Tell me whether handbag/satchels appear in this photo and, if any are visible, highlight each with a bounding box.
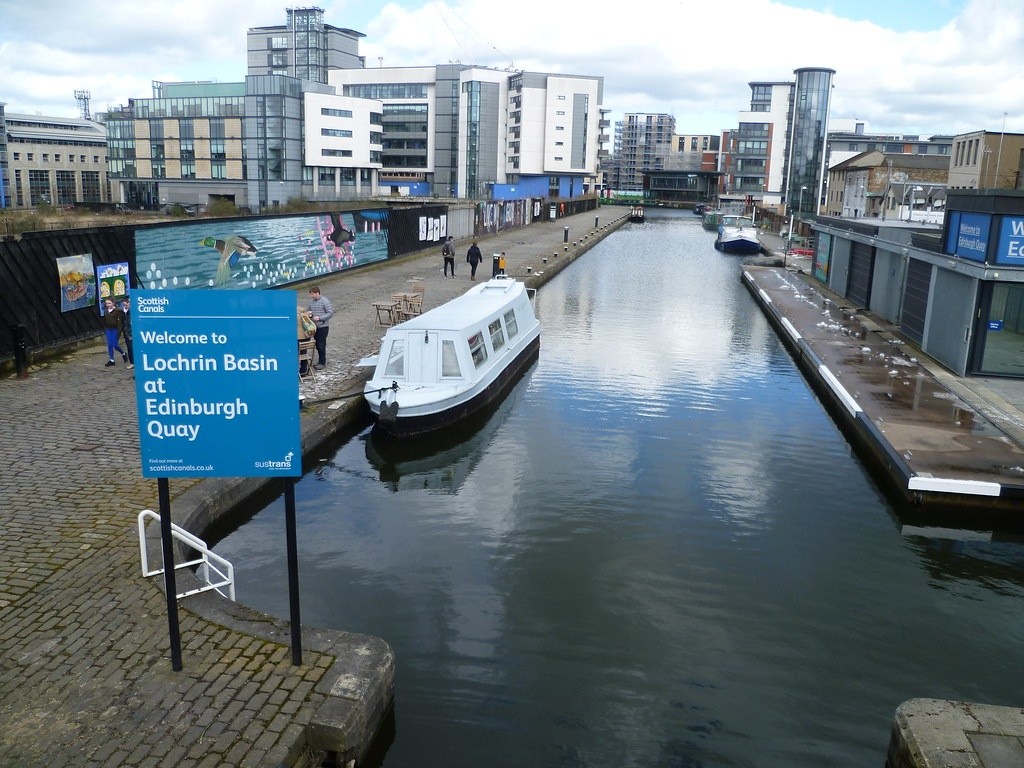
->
[302,314,316,335]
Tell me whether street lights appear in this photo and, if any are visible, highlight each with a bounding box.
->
[799,186,807,213]
[788,210,794,241]
[761,184,766,194]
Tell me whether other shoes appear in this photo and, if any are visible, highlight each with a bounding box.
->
[444,277,448,279]
[127,364,134,369]
[300,372,307,377]
[451,275,456,278]
[122,352,128,362]
[105,361,115,367]
[313,363,326,370]
[471,276,476,281]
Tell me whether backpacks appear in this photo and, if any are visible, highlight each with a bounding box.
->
[442,243,452,256]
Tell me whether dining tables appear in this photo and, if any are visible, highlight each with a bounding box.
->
[372,301,400,331]
[393,293,419,320]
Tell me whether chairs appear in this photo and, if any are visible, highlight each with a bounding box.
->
[299,340,316,384]
[411,286,425,314]
[404,298,423,322]
[388,295,403,325]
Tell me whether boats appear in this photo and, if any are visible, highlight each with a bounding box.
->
[693,203,707,215]
[714,215,762,255]
[364,274,539,439]
[701,206,725,230]
[628,206,645,223]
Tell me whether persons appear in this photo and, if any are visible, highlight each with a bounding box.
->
[297,286,333,377]
[497,251,506,279]
[442,235,457,280]
[466,241,483,281]
[102,295,135,369]
